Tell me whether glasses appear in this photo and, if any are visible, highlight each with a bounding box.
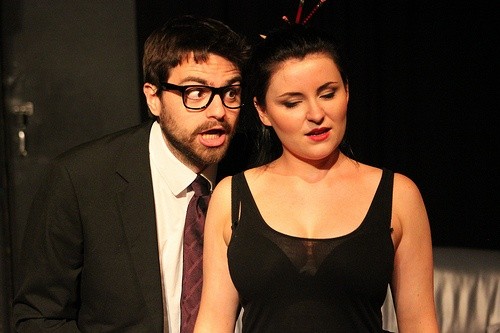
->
[162,80,249,110]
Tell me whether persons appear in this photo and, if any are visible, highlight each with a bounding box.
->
[192,24,437,333]
[8,14,276,333]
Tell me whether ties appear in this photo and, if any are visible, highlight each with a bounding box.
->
[179,175,211,333]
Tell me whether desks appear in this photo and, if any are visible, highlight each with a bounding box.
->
[380,247,500,333]
